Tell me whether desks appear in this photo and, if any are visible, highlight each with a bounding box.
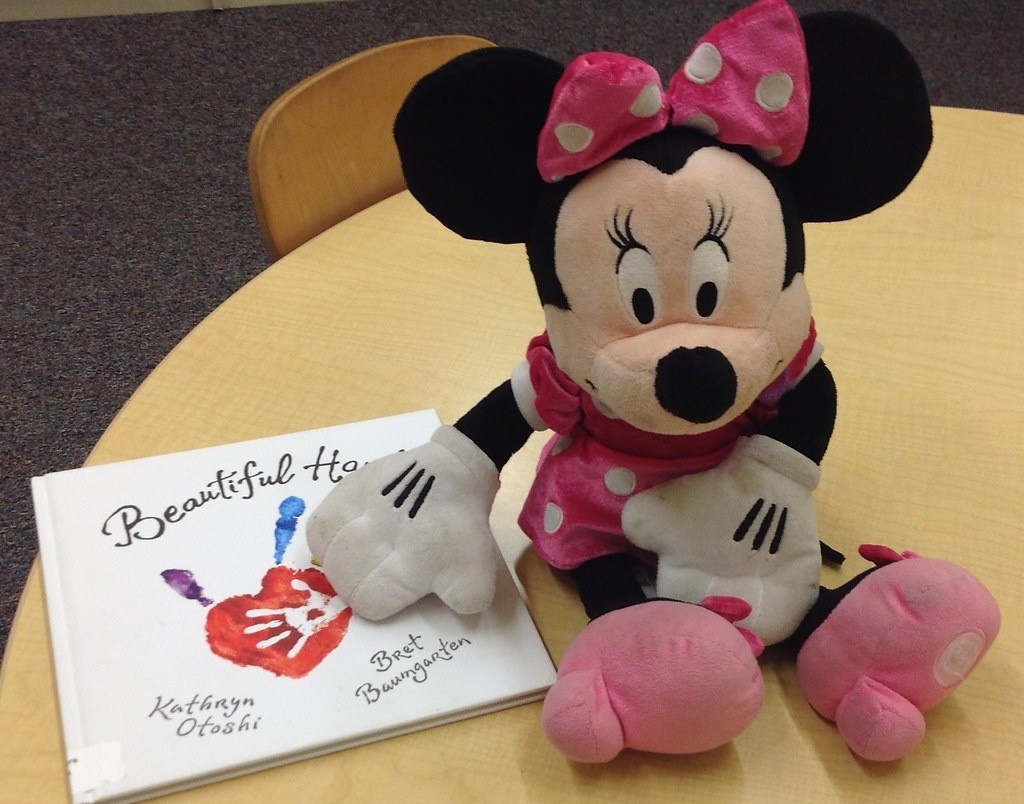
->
[2,101,1024,804]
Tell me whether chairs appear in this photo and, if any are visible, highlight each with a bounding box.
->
[254,34,495,262]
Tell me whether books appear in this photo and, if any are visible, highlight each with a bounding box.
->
[29,409,559,804]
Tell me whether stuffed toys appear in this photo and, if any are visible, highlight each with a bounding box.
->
[307,0,1000,765]
